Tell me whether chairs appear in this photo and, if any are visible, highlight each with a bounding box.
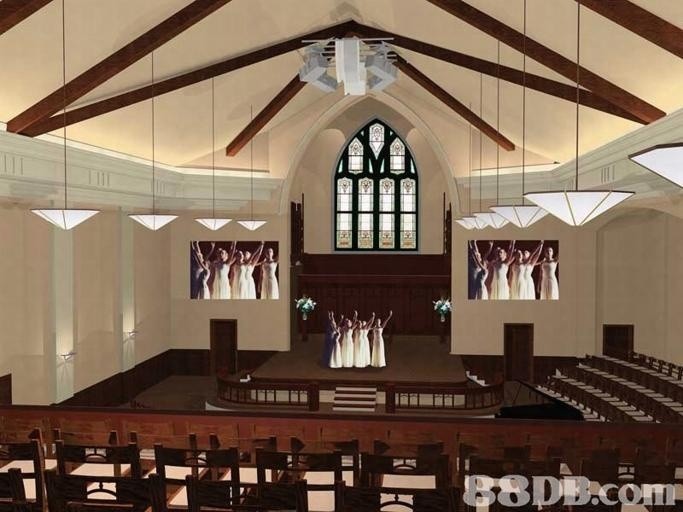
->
[512,348,682,419]
[1,422,681,512]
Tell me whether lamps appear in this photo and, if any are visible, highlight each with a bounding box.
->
[630,143,682,189]
[522,1,636,228]
[298,31,400,101]
[32,1,98,230]
[236,103,267,232]
[193,77,234,231]
[456,40,507,230]
[127,51,179,232]
[488,1,549,229]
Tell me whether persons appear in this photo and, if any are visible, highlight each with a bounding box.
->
[324,309,393,369]
[468,239,559,300]
[190,240,279,300]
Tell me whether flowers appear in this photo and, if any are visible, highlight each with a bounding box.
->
[292,294,318,319]
[431,294,453,319]
